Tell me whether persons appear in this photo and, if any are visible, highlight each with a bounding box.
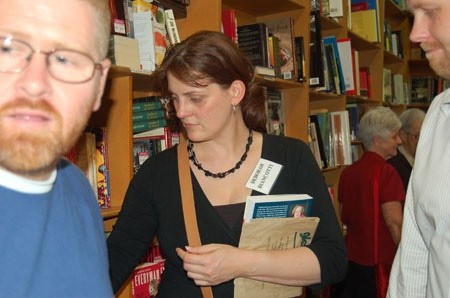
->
[0,0,114,298]
[387,0,450,298]
[337,108,405,298]
[387,108,427,194]
[107,30,348,298]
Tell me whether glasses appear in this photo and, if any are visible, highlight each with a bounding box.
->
[0,35,103,84]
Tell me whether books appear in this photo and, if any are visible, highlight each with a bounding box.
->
[64,0,450,298]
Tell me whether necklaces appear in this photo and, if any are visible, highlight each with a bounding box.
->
[186,128,253,178]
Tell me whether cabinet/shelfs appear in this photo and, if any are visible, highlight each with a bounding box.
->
[62,0,411,298]
[407,12,449,109]
